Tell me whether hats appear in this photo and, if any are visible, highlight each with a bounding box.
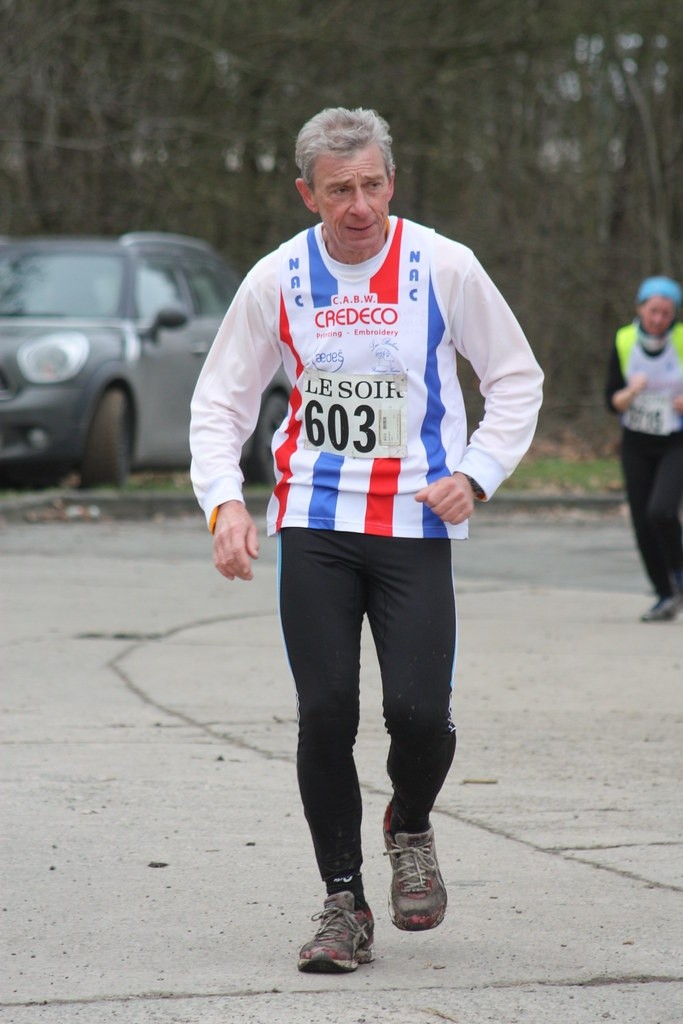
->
[636,275,682,306]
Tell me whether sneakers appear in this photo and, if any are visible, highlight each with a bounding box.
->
[298,891,375,971]
[382,801,447,932]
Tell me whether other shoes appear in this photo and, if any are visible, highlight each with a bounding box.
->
[641,590,683,621]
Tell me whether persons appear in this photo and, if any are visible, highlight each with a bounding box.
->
[189,108,545,973]
[604,277,683,624]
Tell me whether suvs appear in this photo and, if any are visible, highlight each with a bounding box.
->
[1,226,298,494]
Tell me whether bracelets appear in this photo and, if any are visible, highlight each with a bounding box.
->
[467,476,483,496]
